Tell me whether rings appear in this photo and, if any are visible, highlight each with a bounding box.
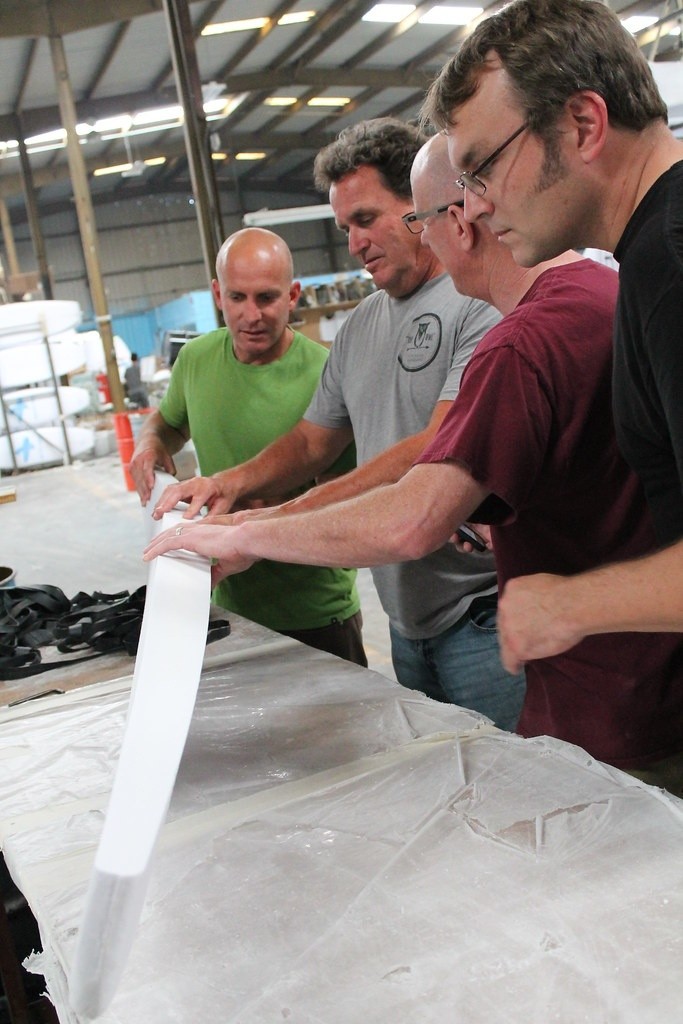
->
[175,526,184,536]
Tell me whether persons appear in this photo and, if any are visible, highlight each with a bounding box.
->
[420,1,683,677]
[127,225,369,669]
[142,129,683,800]
[153,113,526,734]
[123,352,151,410]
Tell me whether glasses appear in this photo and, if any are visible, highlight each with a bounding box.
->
[402,200,464,234]
[454,123,532,196]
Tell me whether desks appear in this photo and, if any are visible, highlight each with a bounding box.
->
[0,463,683,1024]
